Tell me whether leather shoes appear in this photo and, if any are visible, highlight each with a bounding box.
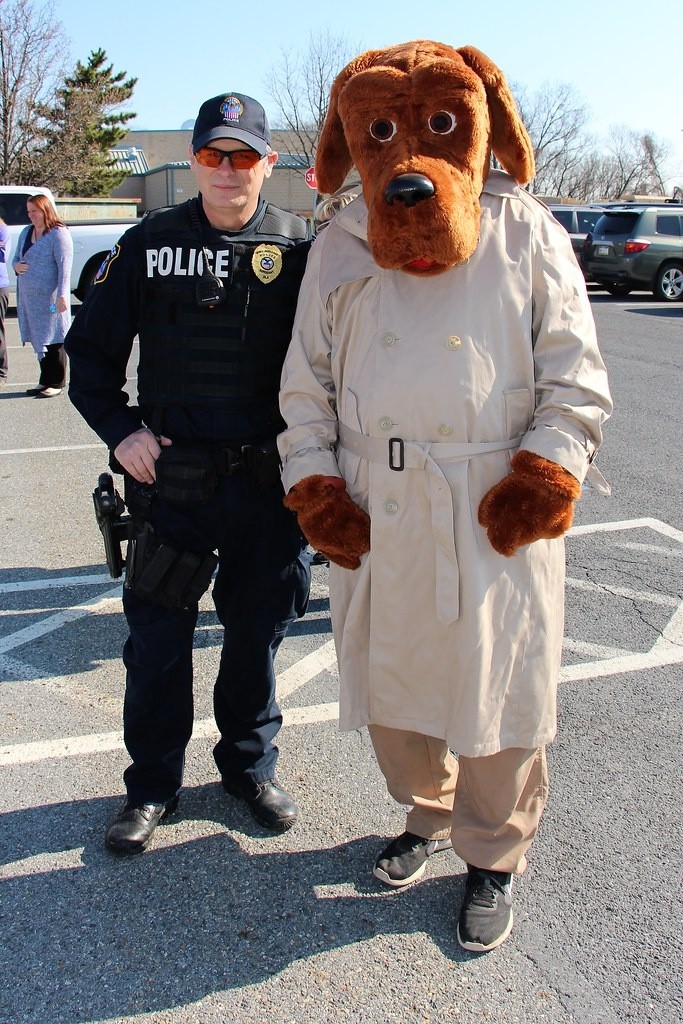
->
[105,789,178,854]
[221,772,300,832]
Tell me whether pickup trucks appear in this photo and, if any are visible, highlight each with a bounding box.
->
[1,185,145,303]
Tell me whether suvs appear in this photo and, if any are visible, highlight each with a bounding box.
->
[544,206,606,282]
[582,202,683,302]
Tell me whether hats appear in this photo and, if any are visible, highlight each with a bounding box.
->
[190,92,271,159]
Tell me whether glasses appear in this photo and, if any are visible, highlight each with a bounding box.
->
[194,145,262,169]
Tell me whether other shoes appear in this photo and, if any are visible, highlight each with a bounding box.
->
[26,384,47,396]
[0,377,7,387]
[39,387,62,398]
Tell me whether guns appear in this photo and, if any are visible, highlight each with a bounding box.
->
[95,472,127,579]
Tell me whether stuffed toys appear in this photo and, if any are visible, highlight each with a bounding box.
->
[278,40,613,953]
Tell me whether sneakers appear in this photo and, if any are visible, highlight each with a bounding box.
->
[457,862,514,951]
[371,831,455,886]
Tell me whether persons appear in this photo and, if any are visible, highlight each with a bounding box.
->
[0,218,10,381]
[62,91,317,851]
[11,194,73,397]
